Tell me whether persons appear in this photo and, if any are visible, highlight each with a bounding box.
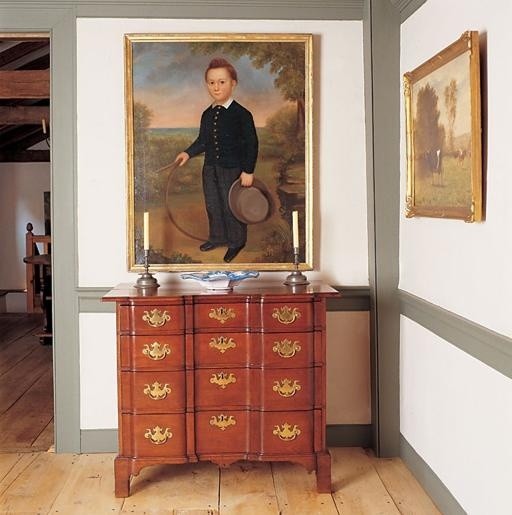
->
[175,56,260,263]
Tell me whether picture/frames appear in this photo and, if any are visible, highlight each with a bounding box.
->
[402,30,484,224]
[123,32,314,272]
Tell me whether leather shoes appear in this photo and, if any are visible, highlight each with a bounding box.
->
[224,245,246,263]
[200,242,225,252]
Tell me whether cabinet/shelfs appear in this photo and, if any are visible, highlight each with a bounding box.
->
[101,282,341,498]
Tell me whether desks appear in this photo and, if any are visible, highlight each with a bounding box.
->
[24,254,52,345]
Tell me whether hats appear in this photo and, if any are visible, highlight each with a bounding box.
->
[229,179,274,225]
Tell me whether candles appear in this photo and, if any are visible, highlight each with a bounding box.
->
[143,211,150,251]
[292,210,299,248]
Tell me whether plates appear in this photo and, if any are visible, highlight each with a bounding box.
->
[179,268,260,291]
